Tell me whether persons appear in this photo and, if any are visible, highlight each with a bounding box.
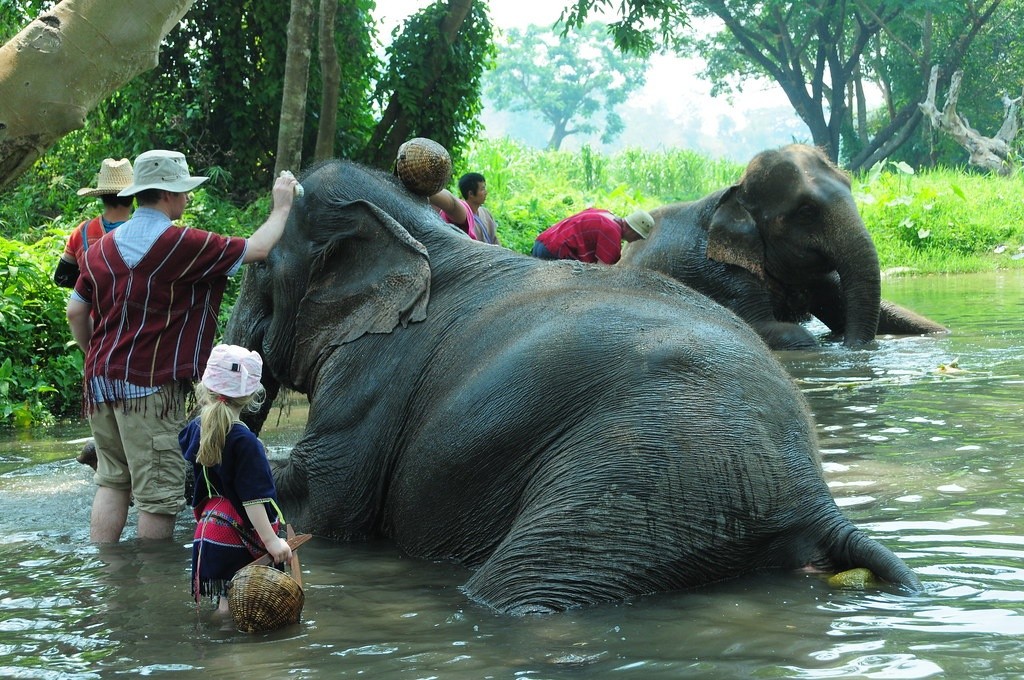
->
[54,159,135,290]
[177,343,293,613]
[427,188,479,243]
[458,172,499,246]
[529,206,655,266]
[67,149,299,542]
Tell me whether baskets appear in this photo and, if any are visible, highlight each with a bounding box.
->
[392,137,452,198]
[224,565,304,634]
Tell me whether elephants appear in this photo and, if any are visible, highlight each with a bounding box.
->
[616,143,948,349]
[76,155,928,620]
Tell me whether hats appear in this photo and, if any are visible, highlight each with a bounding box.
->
[117,150,210,196]
[624,211,655,239]
[202,344,263,398]
[77,158,136,196]
